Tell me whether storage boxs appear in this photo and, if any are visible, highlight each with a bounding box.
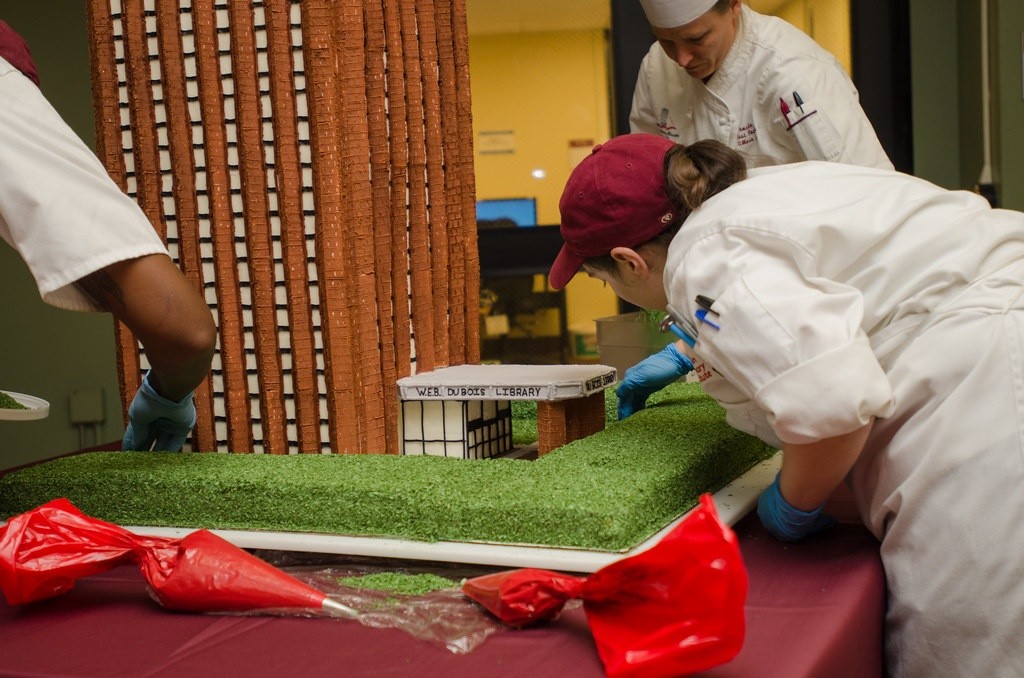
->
[594,310,678,380]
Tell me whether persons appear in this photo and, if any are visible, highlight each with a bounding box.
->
[549,133,1024,678]
[0,22,216,455]
[627,0,896,170]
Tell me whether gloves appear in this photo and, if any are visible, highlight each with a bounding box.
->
[122,369,196,453]
[615,342,694,420]
[756,467,826,538]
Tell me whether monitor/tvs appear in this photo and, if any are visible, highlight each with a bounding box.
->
[475,198,537,226]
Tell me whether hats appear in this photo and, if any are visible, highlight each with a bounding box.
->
[640,0,721,29]
[547,133,689,290]
